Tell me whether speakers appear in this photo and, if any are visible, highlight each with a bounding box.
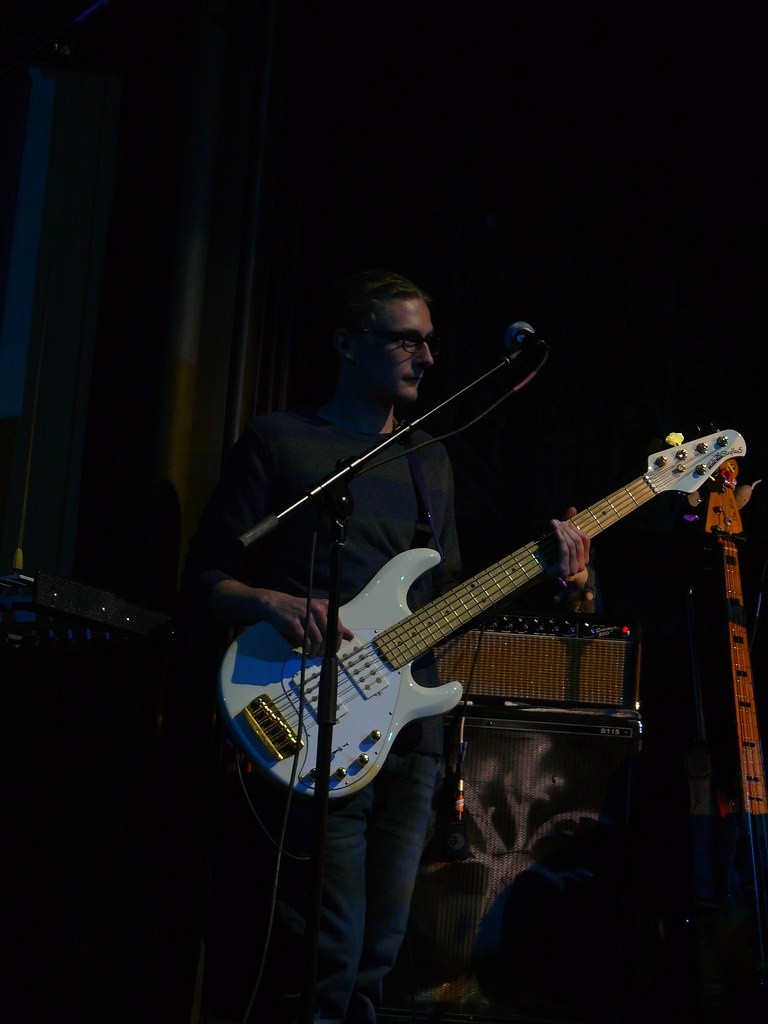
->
[373,713,642,1024]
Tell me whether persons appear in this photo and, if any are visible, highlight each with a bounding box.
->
[184,272,591,1024]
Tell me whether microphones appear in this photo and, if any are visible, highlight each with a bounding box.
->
[504,322,550,353]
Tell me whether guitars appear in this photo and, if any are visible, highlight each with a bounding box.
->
[704,461,767,1024]
[222,415,748,800]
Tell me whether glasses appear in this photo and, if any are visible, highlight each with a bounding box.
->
[349,329,442,356]
